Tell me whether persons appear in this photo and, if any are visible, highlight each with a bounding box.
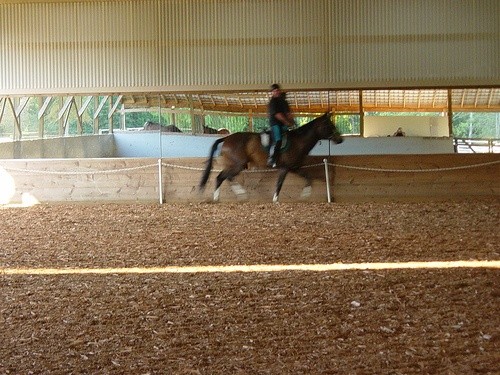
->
[266,82,293,168]
[394,128,405,137]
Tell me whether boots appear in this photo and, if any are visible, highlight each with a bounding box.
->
[266,141,283,169]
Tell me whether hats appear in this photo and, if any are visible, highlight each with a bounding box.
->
[268,83,280,92]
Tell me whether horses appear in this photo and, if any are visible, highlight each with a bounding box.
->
[201,122,230,134]
[197,108,345,206]
[142,120,182,132]
